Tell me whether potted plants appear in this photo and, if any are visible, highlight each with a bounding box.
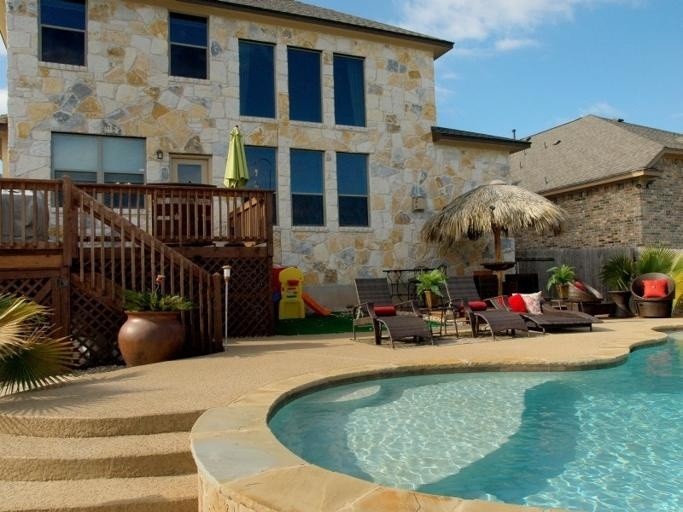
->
[415,269,448,309]
[545,264,576,299]
[596,254,637,318]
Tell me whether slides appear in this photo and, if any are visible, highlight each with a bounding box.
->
[302,292,332,316]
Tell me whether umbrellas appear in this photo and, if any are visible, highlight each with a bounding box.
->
[224,124,251,191]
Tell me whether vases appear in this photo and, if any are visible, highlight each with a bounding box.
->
[118,310,188,366]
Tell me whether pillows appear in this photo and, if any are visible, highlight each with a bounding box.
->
[508,294,528,313]
[457,300,487,311]
[512,291,543,315]
[642,278,667,298]
[373,306,396,316]
[576,281,588,292]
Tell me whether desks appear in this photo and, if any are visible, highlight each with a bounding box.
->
[420,308,459,340]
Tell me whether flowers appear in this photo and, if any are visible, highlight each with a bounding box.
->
[119,275,193,311]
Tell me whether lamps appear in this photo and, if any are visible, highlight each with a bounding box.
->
[157,150,163,159]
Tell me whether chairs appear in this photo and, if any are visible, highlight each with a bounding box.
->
[484,295,604,335]
[631,272,675,317]
[568,280,603,316]
[352,277,435,348]
[444,276,529,341]
